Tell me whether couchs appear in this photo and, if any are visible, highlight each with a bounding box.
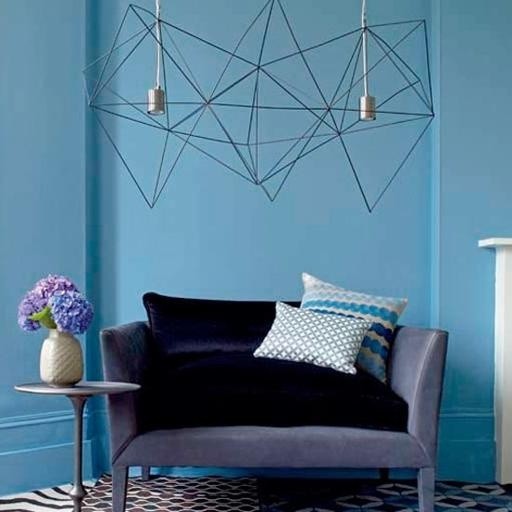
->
[99,318,452,512]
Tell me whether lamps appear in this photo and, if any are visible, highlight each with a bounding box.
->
[358,0,376,122]
[146,0,165,115]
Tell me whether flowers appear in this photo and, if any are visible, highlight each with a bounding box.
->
[16,272,95,335]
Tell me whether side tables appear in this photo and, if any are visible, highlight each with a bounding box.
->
[13,381,142,512]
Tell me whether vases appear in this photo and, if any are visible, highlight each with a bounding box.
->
[40,329,84,387]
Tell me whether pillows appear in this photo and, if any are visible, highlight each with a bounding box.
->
[142,291,301,356]
[299,271,409,382]
[253,302,371,375]
